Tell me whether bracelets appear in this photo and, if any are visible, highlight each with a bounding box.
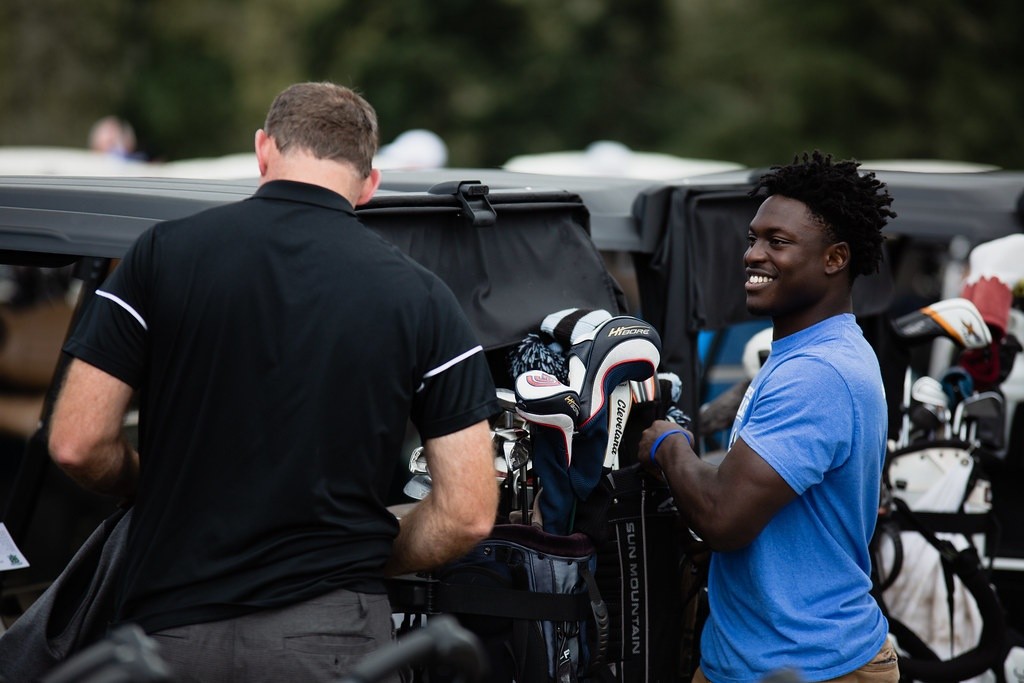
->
[650,429,690,463]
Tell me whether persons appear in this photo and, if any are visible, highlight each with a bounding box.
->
[636,149,902,683]
[86,115,148,162]
[46,81,501,683]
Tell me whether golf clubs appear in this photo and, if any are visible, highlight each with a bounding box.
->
[888,369,1008,455]
[403,426,533,525]
